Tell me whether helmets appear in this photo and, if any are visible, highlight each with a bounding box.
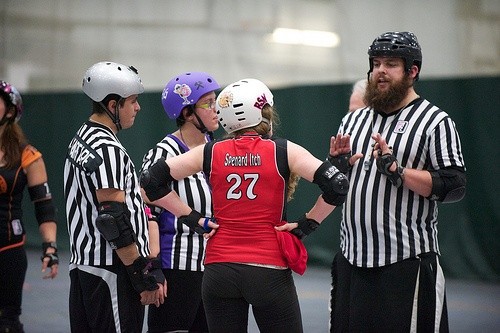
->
[81,62,145,103]
[0,80,24,126]
[162,72,221,120]
[214,79,274,134]
[369,31,422,72]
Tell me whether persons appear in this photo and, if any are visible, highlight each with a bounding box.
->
[139,79,349,333]
[0,80,59,333]
[349,79,368,113]
[327,32,467,333]
[64,61,160,333]
[139,72,221,333]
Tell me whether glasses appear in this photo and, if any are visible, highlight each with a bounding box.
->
[196,99,217,110]
